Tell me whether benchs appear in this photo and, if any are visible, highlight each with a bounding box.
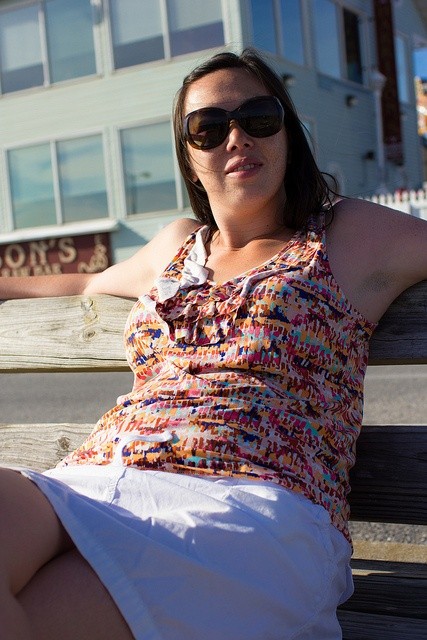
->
[0,281,427,640]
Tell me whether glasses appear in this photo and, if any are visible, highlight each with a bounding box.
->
[180,94,284,151]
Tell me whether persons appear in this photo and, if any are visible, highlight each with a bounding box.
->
[1,47,425,637]
